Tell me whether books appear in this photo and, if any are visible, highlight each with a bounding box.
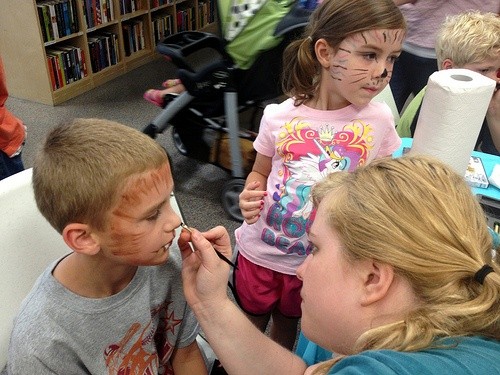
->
[36,0,221,92]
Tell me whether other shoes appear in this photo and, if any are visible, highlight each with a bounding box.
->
[144,88,170,106]
[163,78,182,87]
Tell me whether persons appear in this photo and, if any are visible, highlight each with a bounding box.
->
[217,0,499,157]
[0,57,26,182]
[143,79,187,106]
[7,118,210,375]
[217,0,404,375]
[178,154,500,375]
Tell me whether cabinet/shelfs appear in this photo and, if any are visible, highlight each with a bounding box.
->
[0,0,219,107]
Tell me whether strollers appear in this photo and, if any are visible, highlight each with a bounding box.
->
[143,10,310,222]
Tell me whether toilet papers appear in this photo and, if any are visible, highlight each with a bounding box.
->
[408,67,497,176]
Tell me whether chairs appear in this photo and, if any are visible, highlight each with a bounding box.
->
[1,167,215,375]
[138,0,315,221]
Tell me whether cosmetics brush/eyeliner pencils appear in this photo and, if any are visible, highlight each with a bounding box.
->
[180,222,240,270]
